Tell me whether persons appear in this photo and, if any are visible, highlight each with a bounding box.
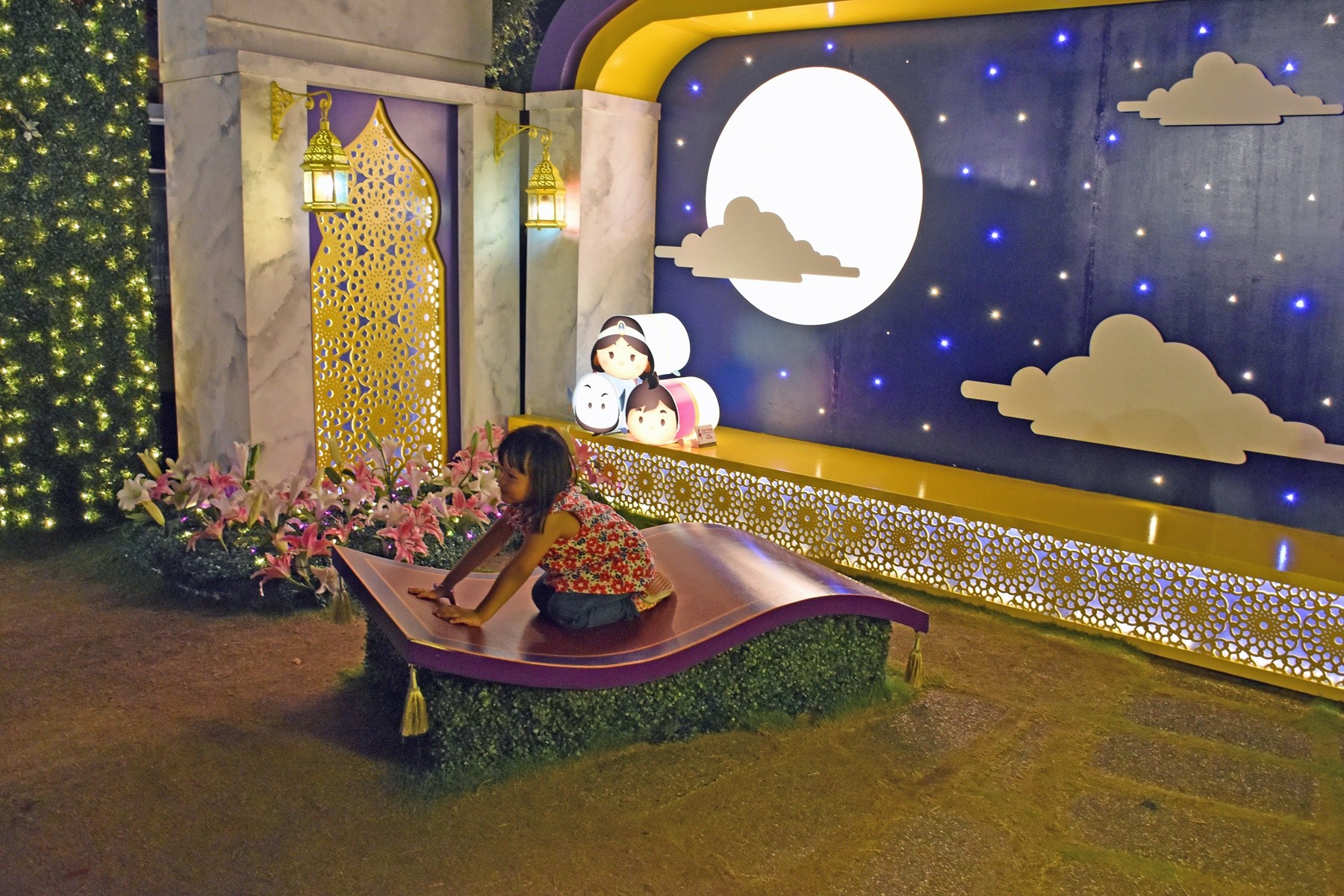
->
[407,424,674,636]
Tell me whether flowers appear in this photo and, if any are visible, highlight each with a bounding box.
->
[118,424,611,609]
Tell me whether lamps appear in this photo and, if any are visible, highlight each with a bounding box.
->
[492,112,567,230]
[269,80,351,213]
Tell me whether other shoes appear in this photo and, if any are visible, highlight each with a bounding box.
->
[630,571,673,612]
